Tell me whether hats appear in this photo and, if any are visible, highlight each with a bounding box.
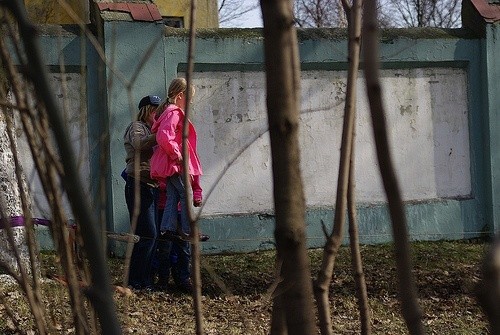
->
[138,95,165,109]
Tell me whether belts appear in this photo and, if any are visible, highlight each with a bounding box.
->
[126,176,135,181]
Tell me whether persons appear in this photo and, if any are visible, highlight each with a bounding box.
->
[121,95,163,289]
[149,77,210,242]
[154,175,203,294]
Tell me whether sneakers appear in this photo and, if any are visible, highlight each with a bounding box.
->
[183,232,209,240]
[174,278,205,296]
[161,230,182,240]
